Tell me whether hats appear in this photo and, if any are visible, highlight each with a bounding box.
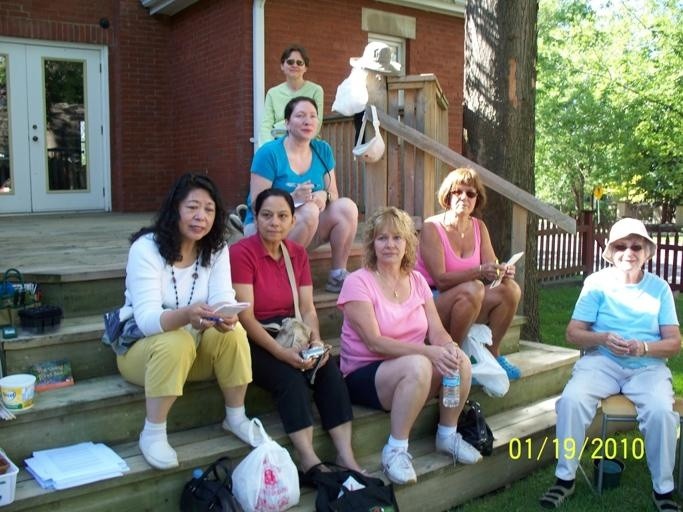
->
[349,42,402,75]
[602,218,657,267]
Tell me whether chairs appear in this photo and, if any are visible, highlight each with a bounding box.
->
[575,336,683,499]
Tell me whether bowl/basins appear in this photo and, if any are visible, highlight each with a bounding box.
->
[0,374,37,411]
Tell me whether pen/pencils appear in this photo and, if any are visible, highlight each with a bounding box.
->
[287,183,318,188]
[0,401,17,421]
[203,317,224,322]
[496,259,500,275]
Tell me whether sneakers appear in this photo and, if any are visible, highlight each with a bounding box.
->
[324,267,351,294]
[226,201,250,236]
[434,431,485,466]
[361,469,372,478]
[379,442,421,486]
[298,464,336,473]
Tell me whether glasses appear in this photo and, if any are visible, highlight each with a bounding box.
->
[613,242,646,253]
[449,189,478,199]
[282,58,307,65]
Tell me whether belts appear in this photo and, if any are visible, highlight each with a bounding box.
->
[428,284,439,292]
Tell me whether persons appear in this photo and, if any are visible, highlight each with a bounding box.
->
[228,44,325,237]
[241,96,358,293]
[537,216,683,512]
[115,173,273,472]
[414,167,521,382]
[227,187,373,486]
[336,205,484,486]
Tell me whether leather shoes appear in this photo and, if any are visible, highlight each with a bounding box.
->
[136,429,181,472]
[220,415,272,449]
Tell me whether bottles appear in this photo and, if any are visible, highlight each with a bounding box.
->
[443,367,461,408]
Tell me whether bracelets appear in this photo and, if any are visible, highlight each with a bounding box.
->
[444,341,458,347]
[642,341,648,357]
[326,191,331,206]
[479,265,482,279]
[310,341,323,346]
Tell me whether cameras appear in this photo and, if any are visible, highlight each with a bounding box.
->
[301,346,325,360]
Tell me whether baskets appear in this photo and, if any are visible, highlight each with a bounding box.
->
[17,303,65,335]
[0,447,22,509]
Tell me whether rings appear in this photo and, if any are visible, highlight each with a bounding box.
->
[298,359,304,364]
[301,368,305,372]
[231,324,235,331]
[199,318,203,326]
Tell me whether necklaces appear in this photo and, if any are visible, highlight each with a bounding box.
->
[449,221,471,239]
[171,249,200,309]
[376,270,402,298]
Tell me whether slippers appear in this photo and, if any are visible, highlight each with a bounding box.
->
[537,477,578,510]
[492,355,523,381]
[470,376,482,387]
[650,488,683,512]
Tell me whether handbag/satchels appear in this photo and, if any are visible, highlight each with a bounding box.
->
[178,455,247,512]
[312,468,401,512]
[456,397,496,459]
[0,268,44,330]
[349,133,388,166]
[273,316,315,355]
